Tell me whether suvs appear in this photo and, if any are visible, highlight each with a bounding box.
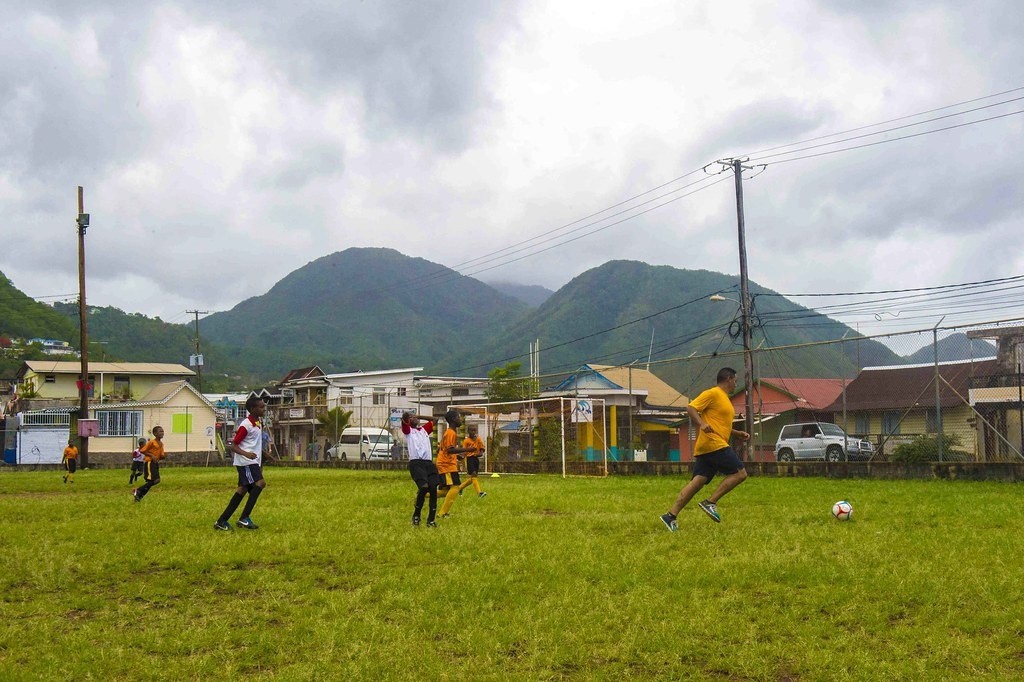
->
[773,422,875,461]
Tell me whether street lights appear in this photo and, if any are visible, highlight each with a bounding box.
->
[709,295,756,462]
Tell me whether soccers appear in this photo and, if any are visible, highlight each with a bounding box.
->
[831,501,853,520]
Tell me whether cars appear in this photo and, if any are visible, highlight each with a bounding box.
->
[326,441,339,461]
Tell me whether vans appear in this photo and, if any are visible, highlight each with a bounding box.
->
[337,427,397,461]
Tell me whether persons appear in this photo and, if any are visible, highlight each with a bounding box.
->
[659,368,751,533]
[62,440,78,483]
[324,439,330,460]
[129,439,146,484]
[460,425,487,498]
[214,397,266,530]
[434,411,478,519]
[403,413,439,527]
[133,426,166,500]
[306,439,319,460]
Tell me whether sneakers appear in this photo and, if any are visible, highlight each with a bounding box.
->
[236,517,259,529]
[213,520,233,531]
[698,499,721,523]
[658,513,677,531]
[133,488,143,501]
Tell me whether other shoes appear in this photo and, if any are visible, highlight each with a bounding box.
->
[427,521,436,527]
[135,474,137,481]
[63,476,66,483]
[412,508,421,525]
[130,478,132,484]
[436,513,449,518]
[478,491,487,498]
